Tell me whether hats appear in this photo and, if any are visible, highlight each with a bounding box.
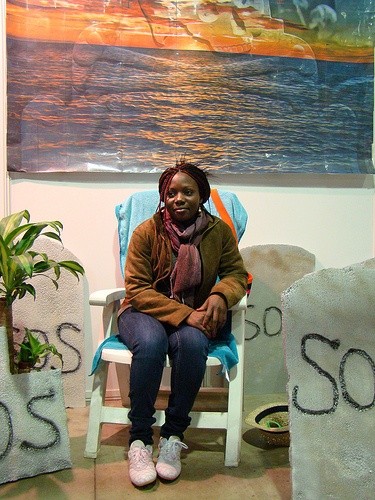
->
[245,401,290,446]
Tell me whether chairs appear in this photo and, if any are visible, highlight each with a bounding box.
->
[84,190,252,467]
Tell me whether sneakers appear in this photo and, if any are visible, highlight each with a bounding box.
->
[127,440,157,486]
[155,435,189,480]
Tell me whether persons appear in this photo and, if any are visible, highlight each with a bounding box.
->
[118,163,249,486]
